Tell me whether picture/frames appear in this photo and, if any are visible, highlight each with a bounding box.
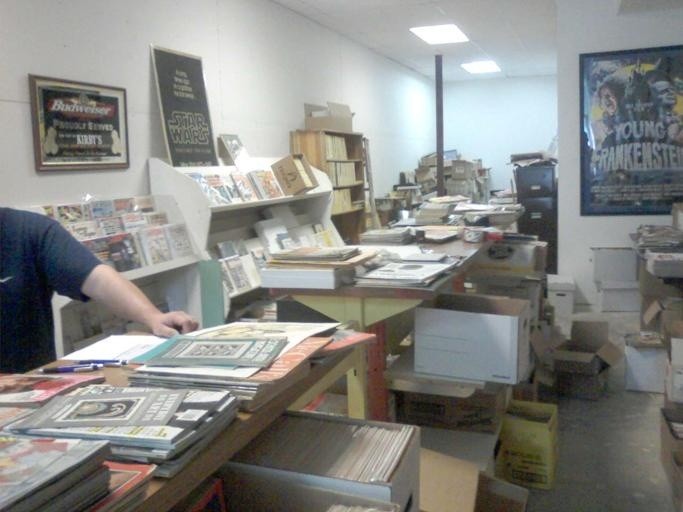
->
[150,42,220,170]
[27,72,132,173]
[577,43,683,217]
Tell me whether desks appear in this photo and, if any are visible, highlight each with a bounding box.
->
[269,192,526,421]
[0,314,371,512]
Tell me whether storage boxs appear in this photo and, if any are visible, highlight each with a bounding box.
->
[451,159,474,181]
[622,328,668,396]
[598,281,643,312]
[658,405,682,475]
[446,179,478,197]
[514,163,558,236]
[586,246,637,285]
[222,405,423,512]
[667,451,683,511]
[553,318,624,401]
[385,372,478,431]
[410,290,532,386]
[271,152,319,196]
[545,273,576,318]
[501,401,563,492]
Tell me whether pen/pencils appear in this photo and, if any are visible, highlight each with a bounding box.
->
[38,364,104,373]
[72,359,128,367]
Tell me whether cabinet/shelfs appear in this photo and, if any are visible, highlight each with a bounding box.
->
[148,149,345,323]
[13,193,227,362]
[290,126,367,247]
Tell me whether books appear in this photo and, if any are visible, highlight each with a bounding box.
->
[183,157,332,203]
[174,477,227,512]
[232,411,414,483]
[324,163,356,185]
[330,188,353,215]
[358,227,412,246]
[0,367,105,407]
[1,430,159,512]
[0,403,35,429]
[415,202,451,225]
[33,193,194,274]
[324,135,348,160]
[209,203,335,297]
[127,318,379,412]
[355,260,459,288]
[16,384,239,479]
[259,244,383,292]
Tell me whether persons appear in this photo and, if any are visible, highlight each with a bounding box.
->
[0,205,199,373]
[653,69,683,145]
[598,79,626,126]
